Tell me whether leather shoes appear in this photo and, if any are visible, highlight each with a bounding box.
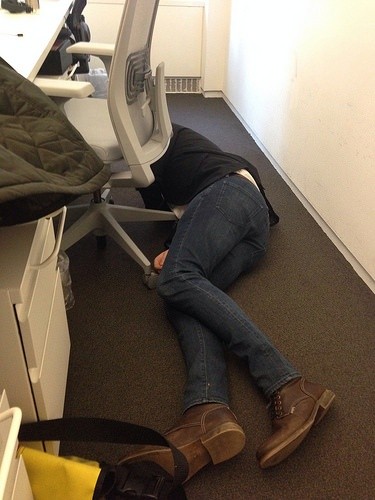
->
[256,375,335,469]
[118,402,247,484]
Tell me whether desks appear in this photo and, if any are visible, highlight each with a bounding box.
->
[0,0,86,110]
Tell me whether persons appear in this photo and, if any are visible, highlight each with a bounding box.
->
[113,121,337,486]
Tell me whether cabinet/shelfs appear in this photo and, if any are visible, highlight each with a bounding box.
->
[0,387,38,500]
[0,206,70,463]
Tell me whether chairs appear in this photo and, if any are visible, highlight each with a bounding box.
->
[26,0,175,296]
